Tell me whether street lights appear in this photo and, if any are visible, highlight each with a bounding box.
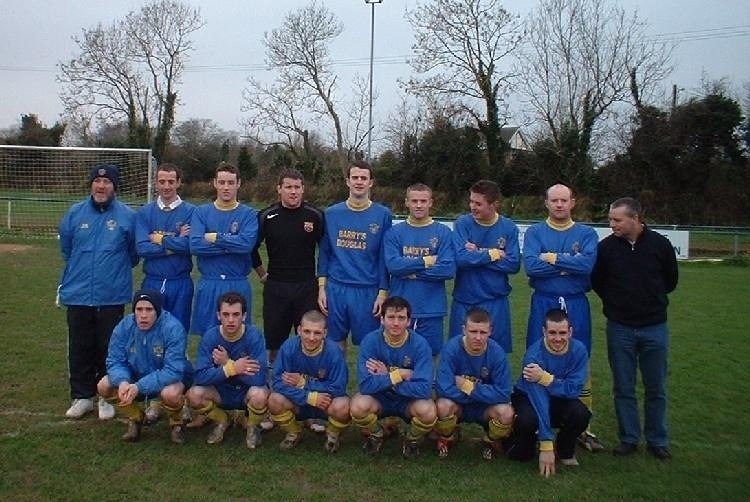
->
[365,0,383,168]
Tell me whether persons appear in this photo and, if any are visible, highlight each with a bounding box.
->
[95,289,195,446]
[254,168,326,434]
[449,179,521,354]
[57,164,139,422]
[316,161,393,354]
[135,160,197,425]
[502,309,591,478]
[349,295,438,462]
[188,163,260,340]
[268,307,352,454]
[184,291,271,450]
[522,183,604,453]
[383,181,457,400]
[433,307,516,460]
[592,197,679,460]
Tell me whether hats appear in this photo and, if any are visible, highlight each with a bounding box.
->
[133,289,160,318]
[89,165,119,191]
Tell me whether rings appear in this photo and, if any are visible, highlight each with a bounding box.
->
[247,367,251,372]
[374,368,377,373]
